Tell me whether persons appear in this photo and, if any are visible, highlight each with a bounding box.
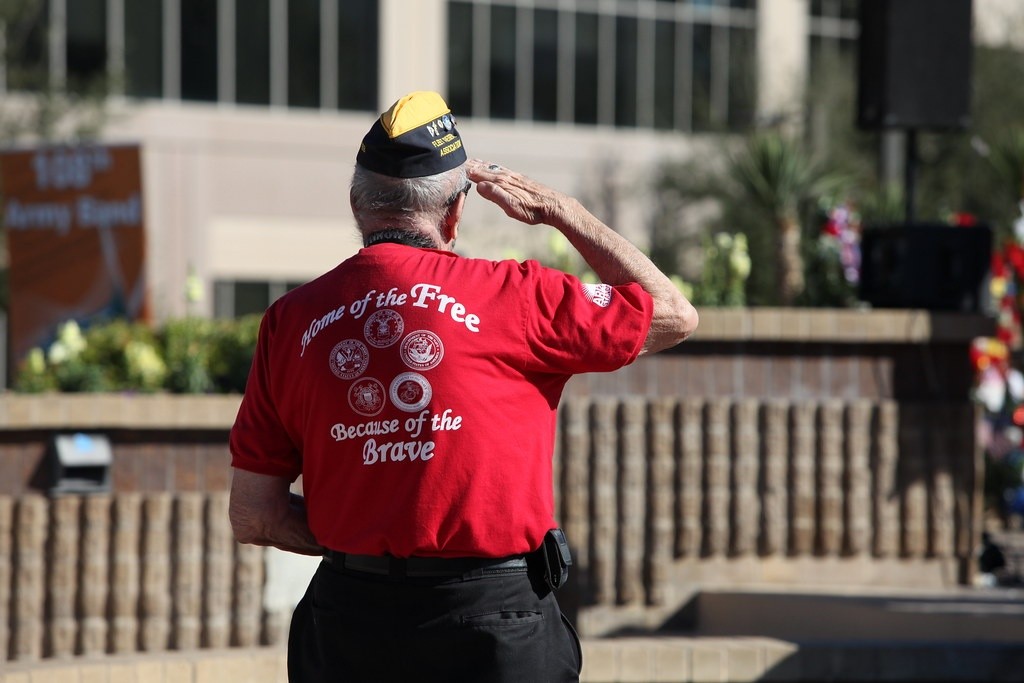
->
[227,91,699,683]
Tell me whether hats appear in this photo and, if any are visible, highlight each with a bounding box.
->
[357,90,468,177]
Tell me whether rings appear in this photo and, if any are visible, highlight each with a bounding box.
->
[488,163,501,172]
